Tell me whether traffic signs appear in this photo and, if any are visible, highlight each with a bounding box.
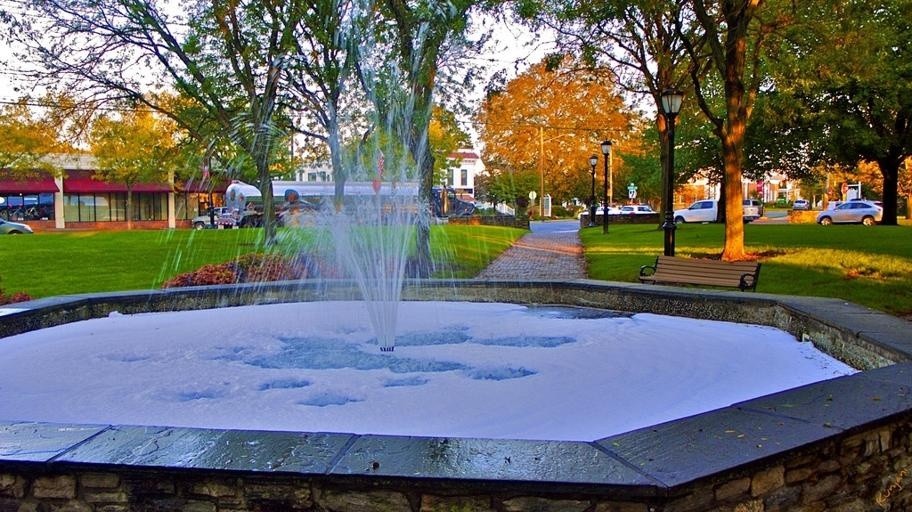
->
[627,186,638,190]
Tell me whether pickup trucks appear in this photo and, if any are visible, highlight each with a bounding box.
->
[675,200,761,222]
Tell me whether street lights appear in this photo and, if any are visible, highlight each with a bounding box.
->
[599,138,612,235]
[660,83,685,255]
[538,118,548,219]
[589,154,599,229]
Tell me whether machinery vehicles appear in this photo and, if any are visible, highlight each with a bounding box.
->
[216,180,482,229]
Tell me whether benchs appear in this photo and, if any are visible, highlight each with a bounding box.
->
[636,252,762,290]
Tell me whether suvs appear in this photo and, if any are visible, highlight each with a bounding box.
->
[192,206,229,230]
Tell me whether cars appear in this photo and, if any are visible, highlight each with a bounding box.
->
[742,199,763,215]
[577,206,620,220]
[620,205,658,214]
[815,200,884,225]
[793,200,809,210]
[0,194,84,220]
[101,207,125,222]
[0,216,36,235]
